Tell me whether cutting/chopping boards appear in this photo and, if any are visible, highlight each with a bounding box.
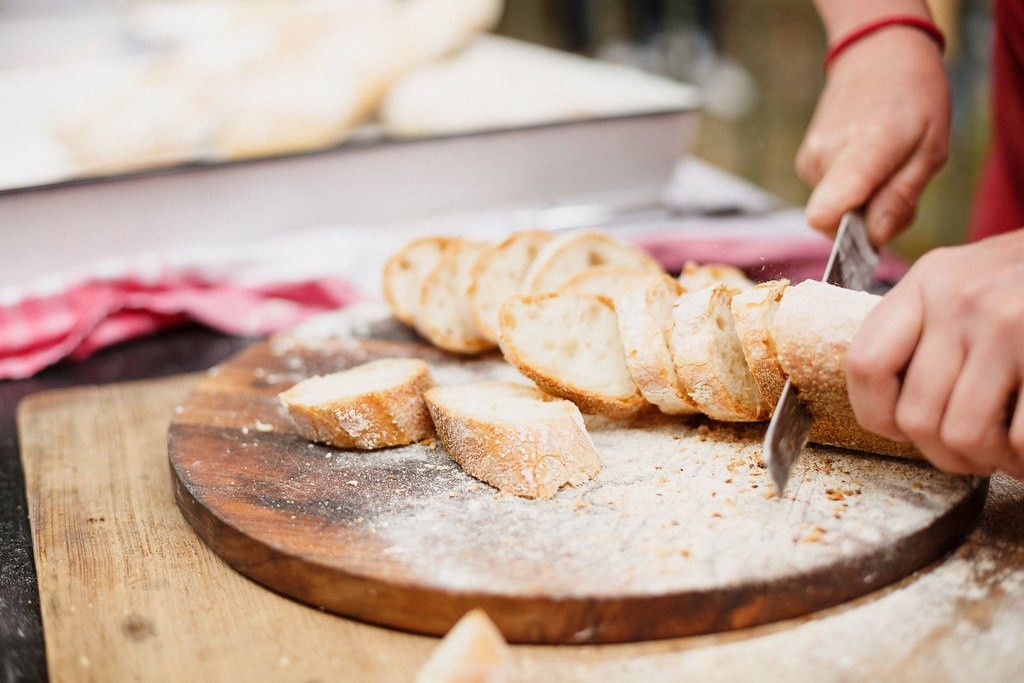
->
[166,296,987,645]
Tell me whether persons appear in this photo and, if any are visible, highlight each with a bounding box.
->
[797,0,1024,476]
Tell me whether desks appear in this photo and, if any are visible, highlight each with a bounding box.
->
[0,279,894,683]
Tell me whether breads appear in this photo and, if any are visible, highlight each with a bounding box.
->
[280,357,440,450]
[772,279,928,458]
[382,229,792,422]
[423,381,603,502]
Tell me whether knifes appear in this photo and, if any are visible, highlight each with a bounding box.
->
[760,207,882,494]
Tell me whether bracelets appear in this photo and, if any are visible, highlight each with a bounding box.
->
[821,15,948,73]
